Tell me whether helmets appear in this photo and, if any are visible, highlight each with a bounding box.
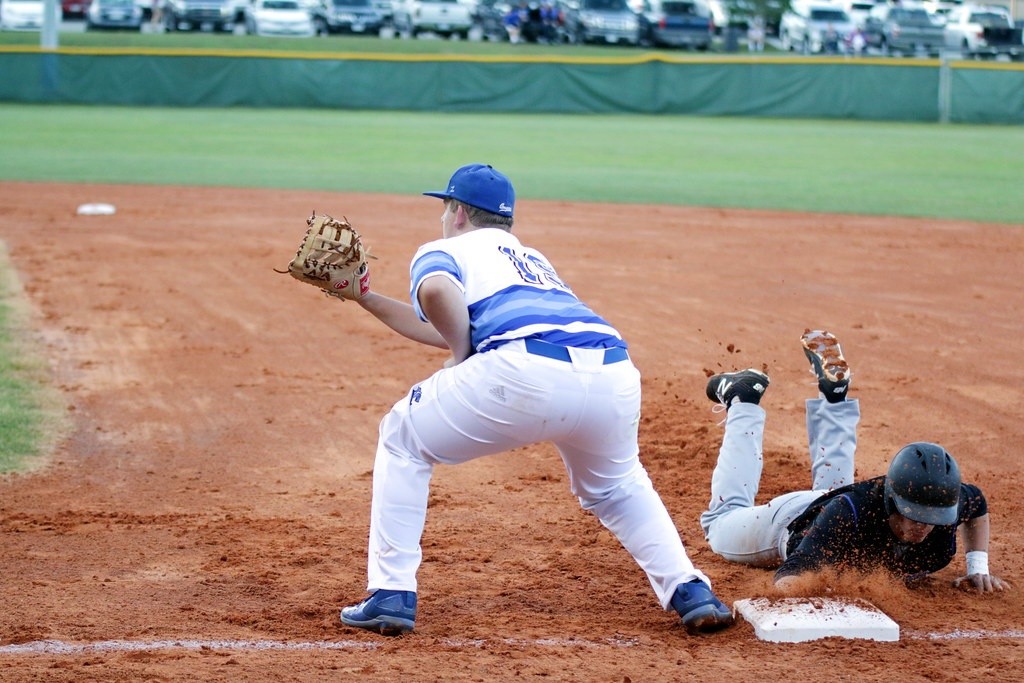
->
[884,441,961,526]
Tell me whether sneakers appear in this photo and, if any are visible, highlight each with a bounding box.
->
[340,589,417,634]
[706,369,770,426]
[800,330,852,404]
[670,581,734,633]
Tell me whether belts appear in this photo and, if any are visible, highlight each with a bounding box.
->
[524,337,628,365]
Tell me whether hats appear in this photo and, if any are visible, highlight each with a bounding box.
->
[422,164,515,217]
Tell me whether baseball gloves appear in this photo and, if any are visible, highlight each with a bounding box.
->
[286,214,372,302]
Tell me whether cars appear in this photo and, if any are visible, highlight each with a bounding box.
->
[0,0,47,31]
[58,0,1024,62]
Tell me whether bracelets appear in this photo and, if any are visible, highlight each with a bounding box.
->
[966,551,989,575]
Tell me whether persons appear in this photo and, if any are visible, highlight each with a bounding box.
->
[287,161,734,634]
[700,325,1013,599]
